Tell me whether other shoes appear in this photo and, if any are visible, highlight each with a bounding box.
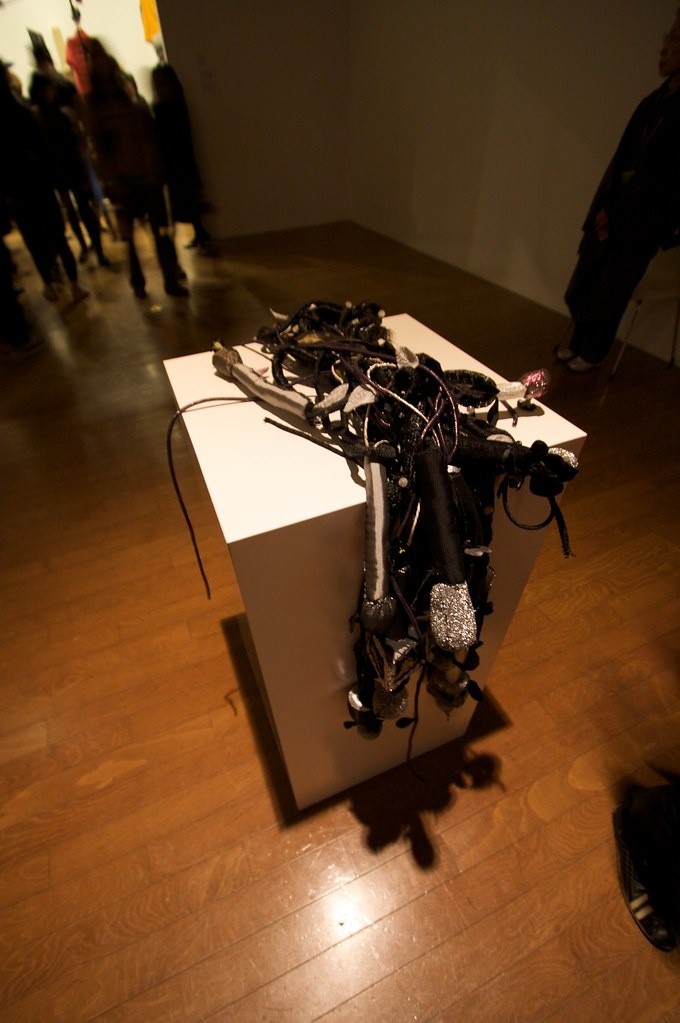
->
[567,356,604,371]
[73,286,89,298]
[614,800,677,952]
[165,280,190,298]
[134,284,147,298]
[557,345,575,361]
[44,289,59,301]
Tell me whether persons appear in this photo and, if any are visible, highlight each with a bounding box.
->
[552,6,680,373]
[0,3,210,361]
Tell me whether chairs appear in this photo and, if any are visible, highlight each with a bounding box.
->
[550,250,680,381]
